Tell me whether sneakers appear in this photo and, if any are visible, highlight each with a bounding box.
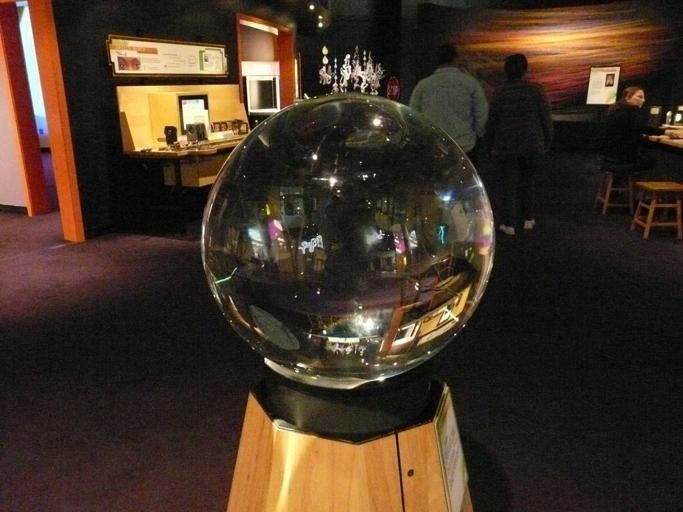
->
[499,219,535,235]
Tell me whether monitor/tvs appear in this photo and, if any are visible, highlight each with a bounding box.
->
[586,63,620,107]
[246,76,281,114]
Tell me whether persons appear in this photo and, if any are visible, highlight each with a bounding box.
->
[600,76,683,208]
[406,41,493,176]
[489,53,554,237]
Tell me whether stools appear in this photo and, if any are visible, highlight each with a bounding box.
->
[591,170,682,241]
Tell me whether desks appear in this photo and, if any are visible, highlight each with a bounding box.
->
[123,132,251,211]
[646,125,682,149]
